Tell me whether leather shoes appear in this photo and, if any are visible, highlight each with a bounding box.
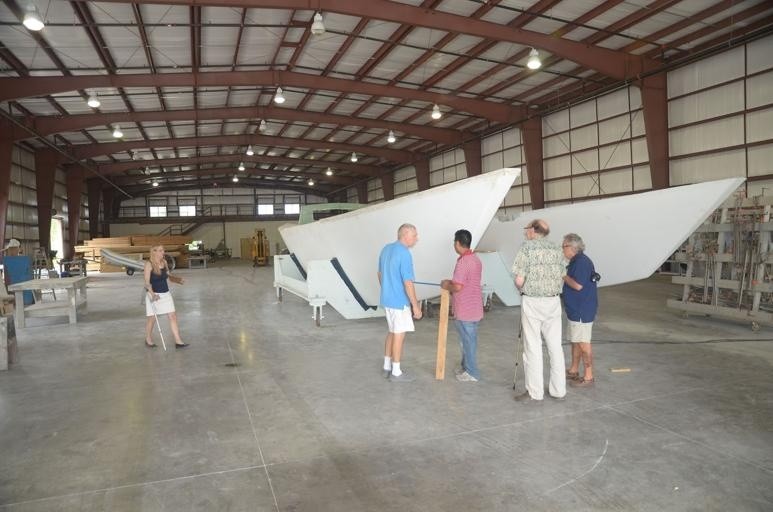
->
[145,341,157,347]
[515,391,541,403]
[175,344,191,350]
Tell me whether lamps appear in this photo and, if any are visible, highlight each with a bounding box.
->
[231,173,238,183]
[144,166,150,175]
[21,0,44,30]
[246,144,253,155]
[113,124,122,138]
[152,177,158,186]
[258,119,267,131]
[273,86,284,103]
[306,178,313,186]
[524,45,541,70]
[310,9,324,36]
[386,129,394,142]
[430,103,441,119]
[350,151,357,162]
[87,95,99,108]
[325,166,332,175]
[237,162,244,170]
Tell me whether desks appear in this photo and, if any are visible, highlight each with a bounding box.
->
[183,255,211,269]
[7,277,88,329]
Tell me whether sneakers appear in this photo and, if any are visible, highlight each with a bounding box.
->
[567,369,594,387]
[381,370,416,382]
[454,367,477,381]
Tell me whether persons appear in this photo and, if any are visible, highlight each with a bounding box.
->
[512,218,567,402]
[563,234,601,387]
[441,229,483,383]
[144,244,190,348]
[377,224,422,381]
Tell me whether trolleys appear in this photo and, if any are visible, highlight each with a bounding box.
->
[83,248,144,276]
[418,284,494,319]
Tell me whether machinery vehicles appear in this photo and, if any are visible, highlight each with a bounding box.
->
[250,227,270,269]
